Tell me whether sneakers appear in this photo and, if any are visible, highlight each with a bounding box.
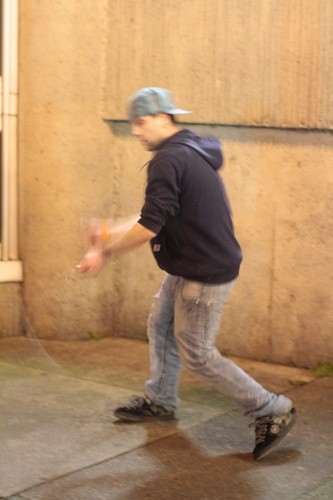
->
[253,406,296,460]
[114,398,174,421]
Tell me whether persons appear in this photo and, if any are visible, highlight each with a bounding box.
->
[71,85,299,460]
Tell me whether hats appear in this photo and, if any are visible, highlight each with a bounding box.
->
[128,87,193,119]
